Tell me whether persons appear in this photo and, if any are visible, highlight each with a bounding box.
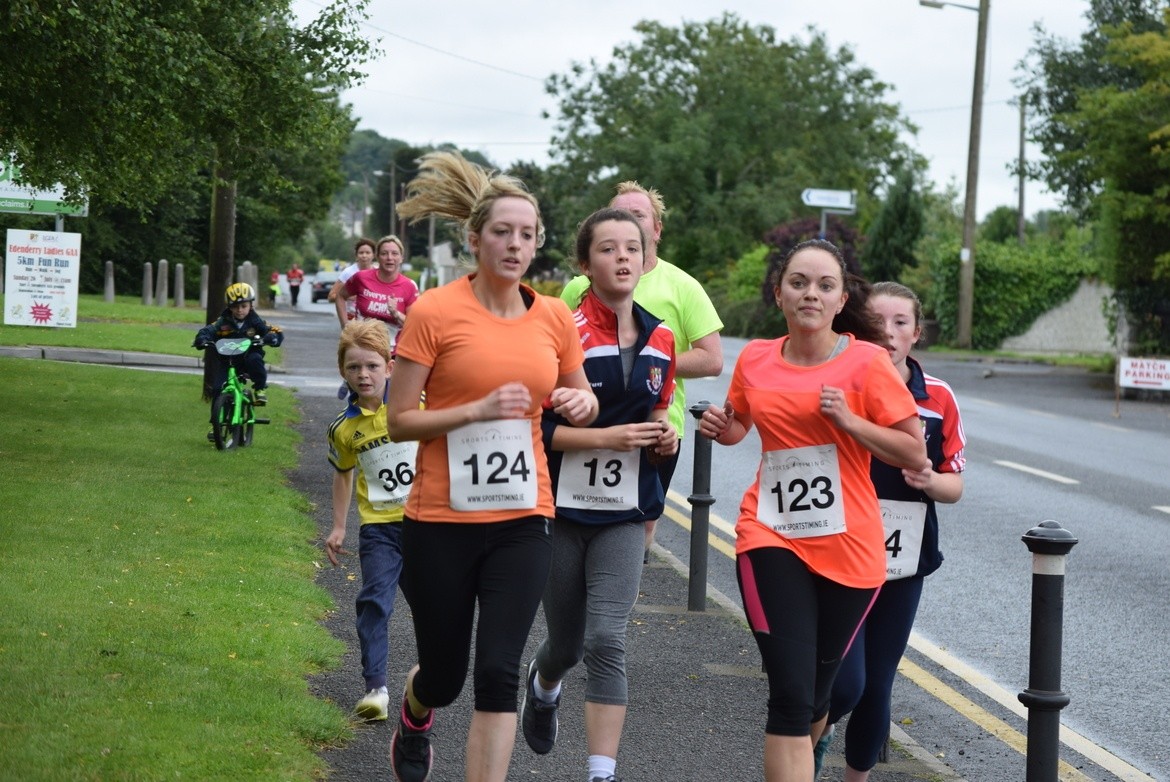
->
[335,233,421,407]
[325,237,380,400]
[195,281,285,442]
[311,314,428,725]
[520,201,681,779]
[375,148,601,781]
[333,256,349,272]
[810,276,969,782]
[541,177,728,566]
[286,263,304,305]
[683,215,932,782]
[269,271,279,304]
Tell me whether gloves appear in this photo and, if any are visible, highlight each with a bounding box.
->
[195,335,209,350]
[264,333,280,348]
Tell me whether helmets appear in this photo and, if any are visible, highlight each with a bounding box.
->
[224,282,256,305]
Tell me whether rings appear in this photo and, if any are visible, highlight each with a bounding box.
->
[707,413,712,422]
[825,399,832,408]
[722,407,735,417]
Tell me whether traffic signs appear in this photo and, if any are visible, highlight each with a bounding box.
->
[1113,355,1170,393]
[801,187,857,209]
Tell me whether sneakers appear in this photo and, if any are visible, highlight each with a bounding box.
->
[390,685,434,782]
[337,382,349,400]
[254,390,267,402]
[356,689,390,723]
[520,657,562,756]
[814,725,836,779]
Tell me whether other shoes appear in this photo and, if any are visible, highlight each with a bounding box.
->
[291,305,296,312]
[269,303,277,309]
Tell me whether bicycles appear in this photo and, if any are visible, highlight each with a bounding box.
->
[196,333,278,454]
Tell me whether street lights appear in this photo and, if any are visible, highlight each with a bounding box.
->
[922,0,993,350]
[413,155,436,250]
[374,168,397,236]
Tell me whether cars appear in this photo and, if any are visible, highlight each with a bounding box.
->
[309,270,345,303]
